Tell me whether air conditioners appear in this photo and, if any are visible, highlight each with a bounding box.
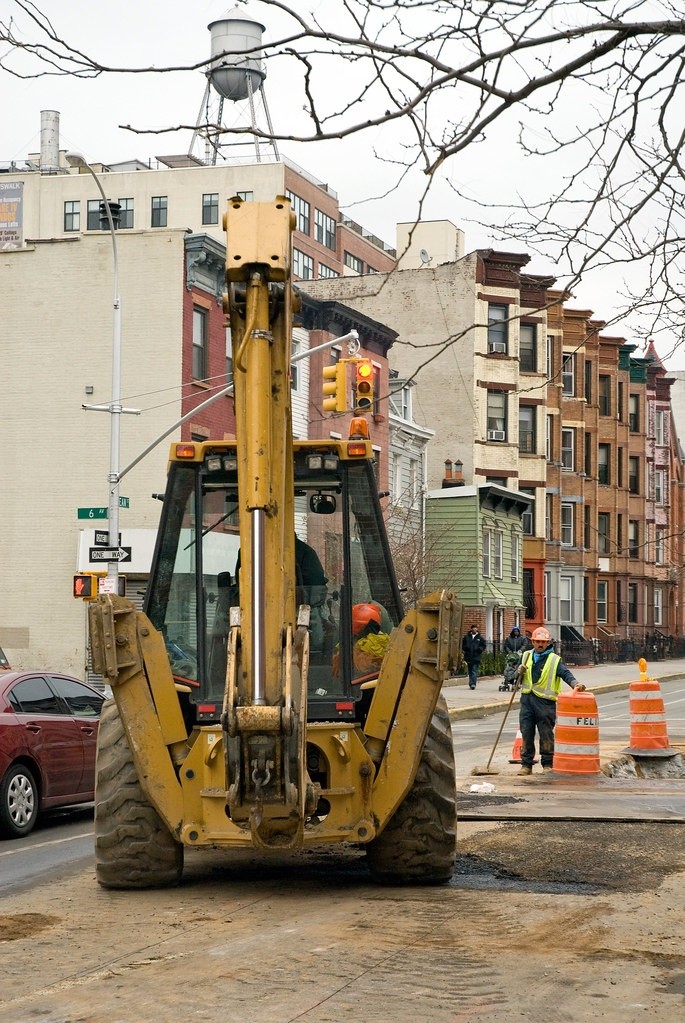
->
[492,431,505,441]
[490,342,505,353]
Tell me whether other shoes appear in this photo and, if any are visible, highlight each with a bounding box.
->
[517,767,532,775]
[471,684,475,690]
[543,766,552,774]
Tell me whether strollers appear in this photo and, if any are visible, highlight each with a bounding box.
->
[498,650,522,692]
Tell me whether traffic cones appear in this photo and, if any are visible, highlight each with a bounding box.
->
[508,723,539,765]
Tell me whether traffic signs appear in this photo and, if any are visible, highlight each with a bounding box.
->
[88,547,131,562]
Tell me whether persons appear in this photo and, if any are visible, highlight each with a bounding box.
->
[641,631,679,662]
[504,627,528,689]
[235,531,329,646]
[514,627,586,776]
[462,625,486,689]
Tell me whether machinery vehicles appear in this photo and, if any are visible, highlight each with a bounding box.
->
[80,186,461,891]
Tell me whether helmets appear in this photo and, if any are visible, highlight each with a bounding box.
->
[352,604,382,635]
[530,627,550,641]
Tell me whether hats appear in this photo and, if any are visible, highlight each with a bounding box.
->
[512,626,519,631]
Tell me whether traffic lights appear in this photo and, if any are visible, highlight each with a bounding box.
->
[118,575,127,597]
[73,574,98,597]
[354,362,374,413]
[322,362,348,413]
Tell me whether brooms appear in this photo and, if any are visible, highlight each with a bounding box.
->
[469,652,531,775]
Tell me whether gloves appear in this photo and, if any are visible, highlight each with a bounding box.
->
[574,683,586,692]
[518,664,528,674]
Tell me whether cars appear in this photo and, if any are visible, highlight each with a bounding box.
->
[0,670,112,840]
